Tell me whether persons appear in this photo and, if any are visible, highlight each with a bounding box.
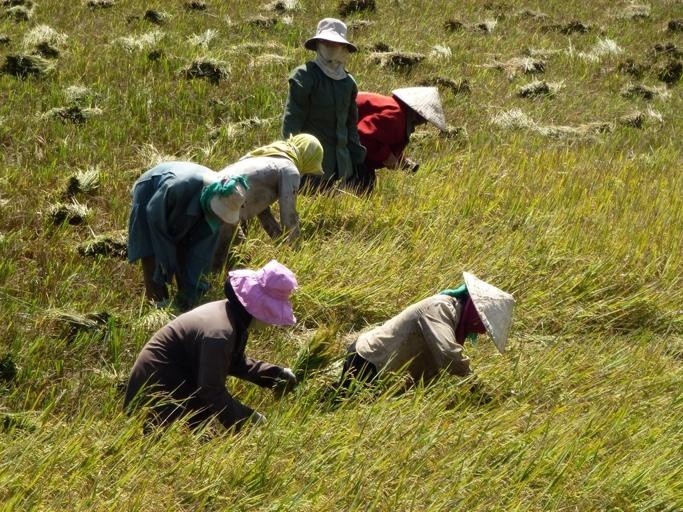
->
[318,273,517,411]
[281,19,368,196]
[128,161,247,312]
[220,133,326,247]
[124,261,297,435]
[356,86,441,194]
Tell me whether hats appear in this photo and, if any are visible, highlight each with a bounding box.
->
[392,86,446,131]
[462,270,514,355]
[204,171,246,224]
[304,18,358,52]
[228,260,296,325]
